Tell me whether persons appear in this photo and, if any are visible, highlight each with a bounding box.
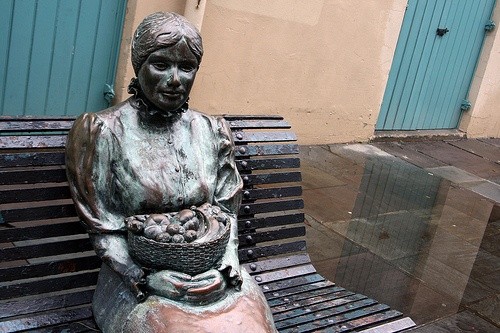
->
[65,10,279,333]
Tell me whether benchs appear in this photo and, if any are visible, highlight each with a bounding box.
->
[0,116,418,333]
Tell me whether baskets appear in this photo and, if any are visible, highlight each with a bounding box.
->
[122,203,232,277]
